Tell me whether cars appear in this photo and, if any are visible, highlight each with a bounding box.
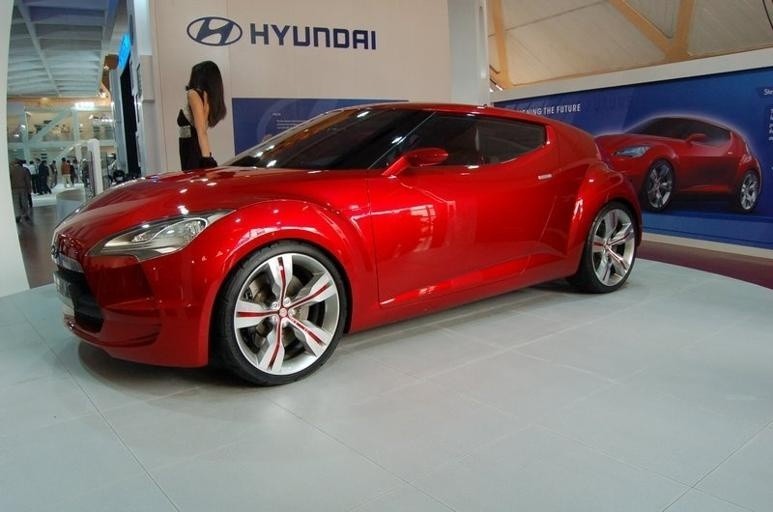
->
[594,116,762,213]
[50,102,642,386]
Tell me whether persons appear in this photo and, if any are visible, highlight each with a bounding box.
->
[176,60,228,171]
[8,157,89,223]
